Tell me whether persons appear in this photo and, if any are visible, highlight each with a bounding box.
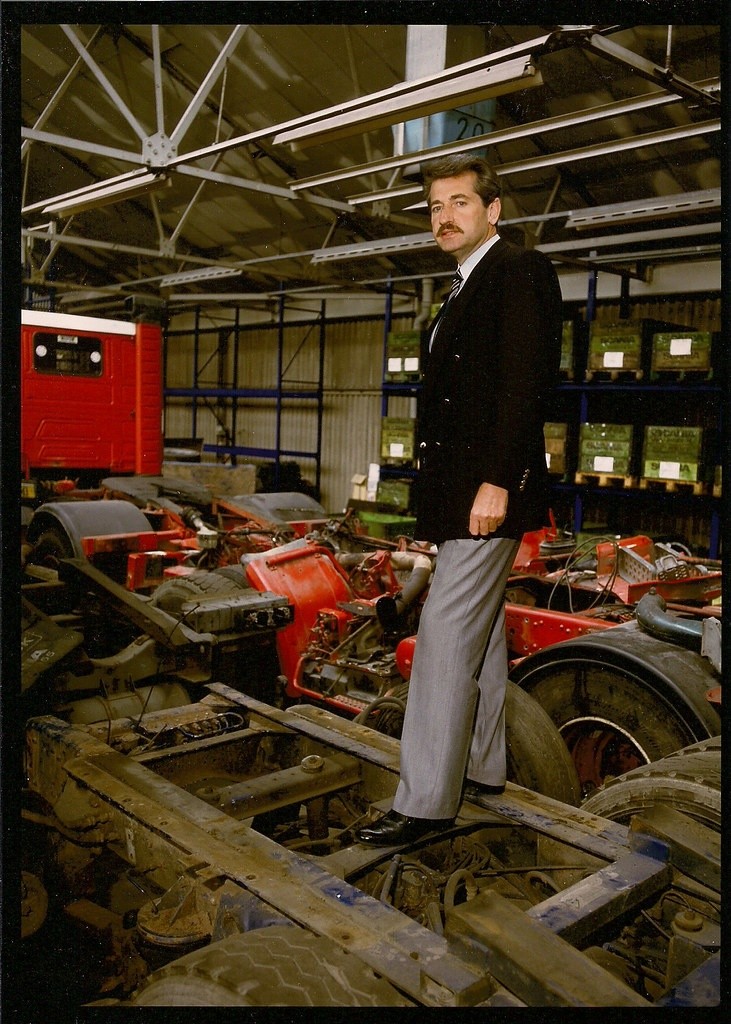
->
[359,151,563,851]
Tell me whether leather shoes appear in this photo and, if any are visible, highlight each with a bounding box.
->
[462,778,505,795]
[355,809,455,845]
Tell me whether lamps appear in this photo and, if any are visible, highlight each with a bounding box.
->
[267,56,544,150]
[40,171,174,220]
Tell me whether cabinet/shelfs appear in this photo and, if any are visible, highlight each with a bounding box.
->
[163,294,328,501]
[382,265,721,561]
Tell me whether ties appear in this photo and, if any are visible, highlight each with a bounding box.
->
[434,268,464,341]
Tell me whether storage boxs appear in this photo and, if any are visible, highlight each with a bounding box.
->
[368,463,419,491]
[350,473,368,501]
